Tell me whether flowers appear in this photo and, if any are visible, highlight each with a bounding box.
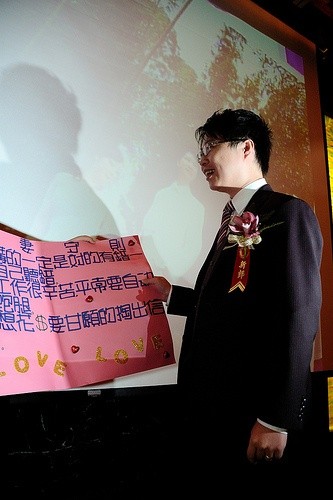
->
[223,212,284,260]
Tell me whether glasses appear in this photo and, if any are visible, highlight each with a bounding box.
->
[196,138,248,164]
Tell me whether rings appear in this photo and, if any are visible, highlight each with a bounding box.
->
[266,456,270,460]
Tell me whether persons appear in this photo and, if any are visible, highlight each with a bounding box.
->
[0,223,108,245]
[141,108,324,500]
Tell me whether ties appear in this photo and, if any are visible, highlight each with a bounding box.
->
[216,199,236,249]
